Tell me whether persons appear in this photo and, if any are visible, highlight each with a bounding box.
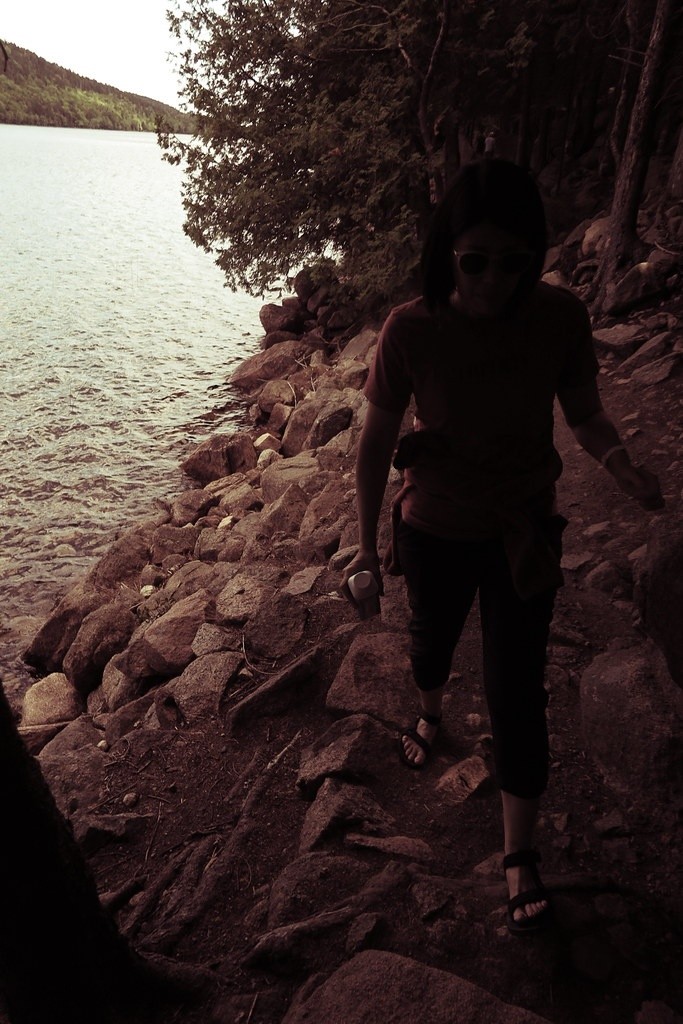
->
[469,128,499,158]
[339,159,667,931]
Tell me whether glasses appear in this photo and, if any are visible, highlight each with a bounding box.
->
[453,246,533,275]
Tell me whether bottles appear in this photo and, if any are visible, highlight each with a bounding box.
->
[348,569,381,621]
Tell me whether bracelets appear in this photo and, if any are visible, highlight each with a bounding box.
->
[601,445,626,468]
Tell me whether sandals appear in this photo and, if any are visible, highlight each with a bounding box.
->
[401,711,443,768]
[503,846,552,935]
